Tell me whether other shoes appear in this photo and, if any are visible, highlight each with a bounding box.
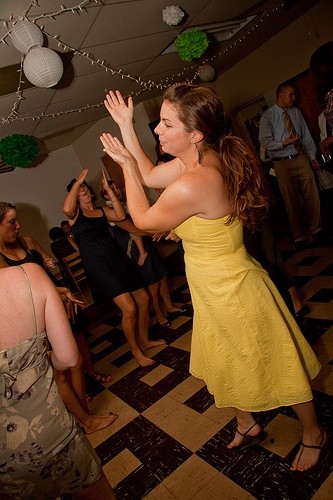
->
[295,229,328,246]
[157,307,188,326]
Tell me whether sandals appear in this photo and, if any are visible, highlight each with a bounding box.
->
[88,372,114,384]
[288,430,331,474]
[221,421,268,454]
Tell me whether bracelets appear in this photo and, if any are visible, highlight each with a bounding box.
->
[106,186,112,194]
[109,194,116,201]
[111,199,120,207]
[63,297,70,305]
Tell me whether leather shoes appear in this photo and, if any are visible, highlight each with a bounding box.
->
[296,304,311,318]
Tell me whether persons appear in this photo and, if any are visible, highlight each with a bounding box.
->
[318,84,333,175]
[49,220,85,284]
[100,84,331,474]
[259,83,325,250]
[99,152,311,327]
[0,263,116,500]
[64,168,166,366]
[0,201,118,435]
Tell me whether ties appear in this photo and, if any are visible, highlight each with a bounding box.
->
[284,113,304,154]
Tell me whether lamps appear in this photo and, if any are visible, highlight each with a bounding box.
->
[11,17,64,89]
[198,64,215,82]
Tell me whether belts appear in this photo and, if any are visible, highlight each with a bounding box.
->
[272,154,300,162]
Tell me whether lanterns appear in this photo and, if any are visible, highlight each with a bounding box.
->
[11,20,43,54]
[23,47,63,88]
[198,64,215,82]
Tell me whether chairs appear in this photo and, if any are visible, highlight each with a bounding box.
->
[62,251,86,293]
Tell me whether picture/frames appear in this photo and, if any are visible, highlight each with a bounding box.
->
[231,93,274,173]
